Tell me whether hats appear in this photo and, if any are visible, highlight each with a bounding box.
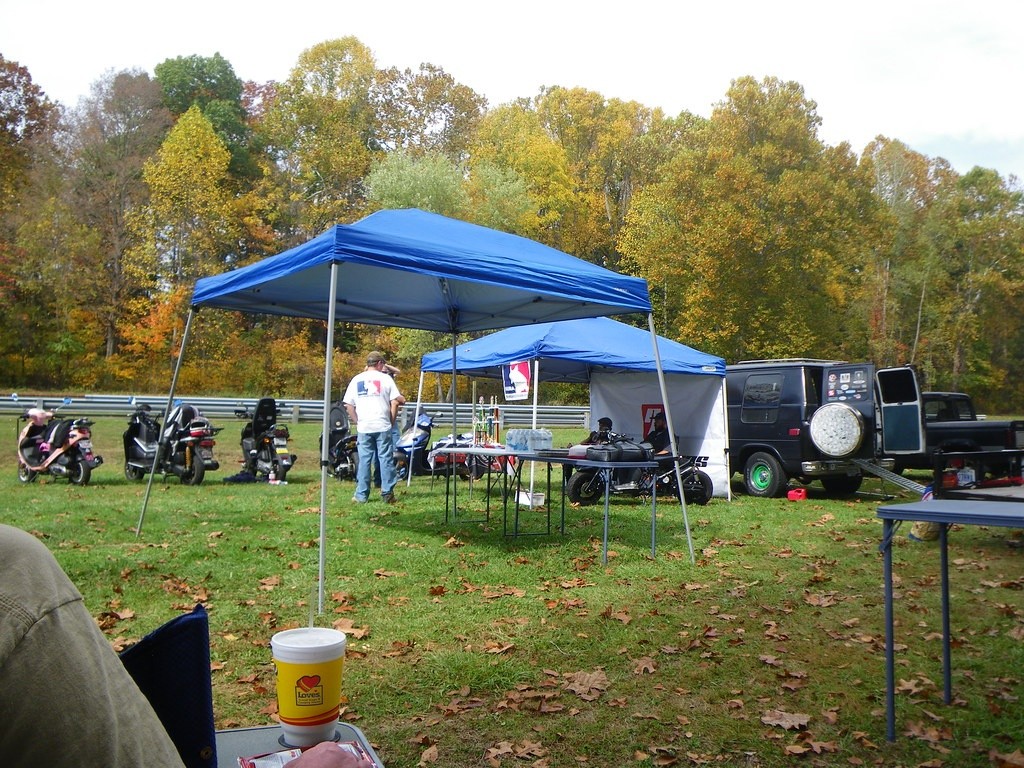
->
[367,351,387,365]
[597,417,612,427]
[650,412,666,420]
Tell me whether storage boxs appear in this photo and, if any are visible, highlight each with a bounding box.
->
[514,490,544,506]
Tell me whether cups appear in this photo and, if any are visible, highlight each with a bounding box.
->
[269,627,348,749]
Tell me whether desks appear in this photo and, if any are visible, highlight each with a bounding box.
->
[427,447,660,566]
[876,484,1024,742]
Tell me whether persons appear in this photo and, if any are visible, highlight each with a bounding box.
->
[342,351,405,504]
[613,412,679,492]
[565,417,612,486]
[0,524,379,768]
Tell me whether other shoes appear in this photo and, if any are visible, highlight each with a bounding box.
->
[352,497,359,503]
[384,494,397,503]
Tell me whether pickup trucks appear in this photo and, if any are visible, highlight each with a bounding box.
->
[894,392,1024,483]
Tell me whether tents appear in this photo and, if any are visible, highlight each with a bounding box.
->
[407,316,732,511]
[135,207,696,613]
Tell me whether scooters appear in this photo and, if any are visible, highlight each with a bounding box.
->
[390,410,485,482]
[12,391,102,487]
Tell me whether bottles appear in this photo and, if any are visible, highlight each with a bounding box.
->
[788,489,806,501]
[505,428,553,452]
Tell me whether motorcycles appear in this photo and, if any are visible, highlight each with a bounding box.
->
[567,428,713,505]
[319,400,359,481]
[234,397,297,481]
[122,396,221,486]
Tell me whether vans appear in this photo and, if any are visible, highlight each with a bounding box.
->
[726,358,928,499]
